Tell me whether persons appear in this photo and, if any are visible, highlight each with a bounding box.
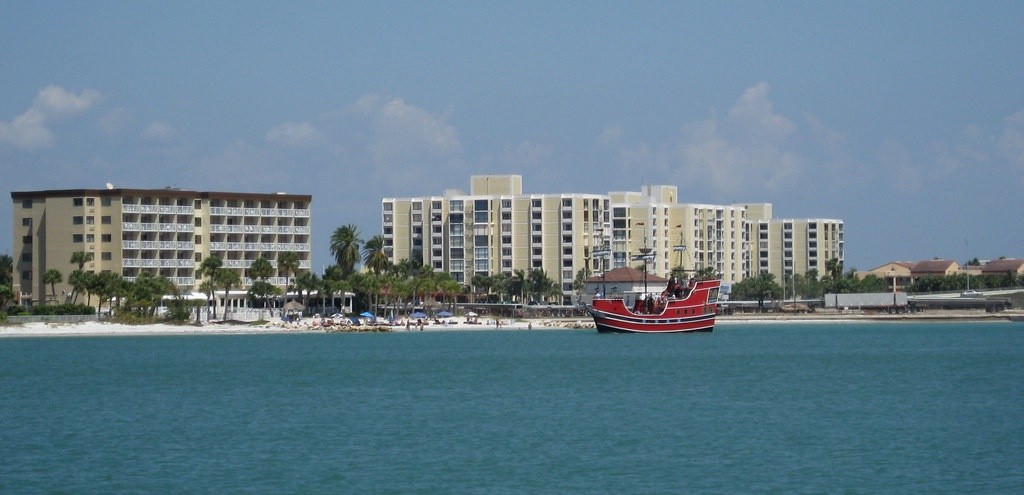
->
[395,318,429,325]
[466,317,495,325]
[496,319,502,328]
[311,317,352,326]
[406,323,423,332]
[363,319,393,326]
[284,317,308,325]
[435,319,453,326]
[528,322,532,330]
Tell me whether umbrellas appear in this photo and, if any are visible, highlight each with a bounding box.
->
[283,301,305,314]
[465,310,478,322]
[331,313,343,325]
[359,311,373,323]
[437,310,454,323]
[409,311,427,323]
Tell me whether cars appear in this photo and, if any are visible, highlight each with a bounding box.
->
[961,290,982,298]
[528,302,538,305]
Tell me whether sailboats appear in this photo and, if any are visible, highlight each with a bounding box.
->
[576,218,722,333]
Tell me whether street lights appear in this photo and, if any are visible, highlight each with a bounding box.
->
[913,276,918,283]
[961,236,969,290]
